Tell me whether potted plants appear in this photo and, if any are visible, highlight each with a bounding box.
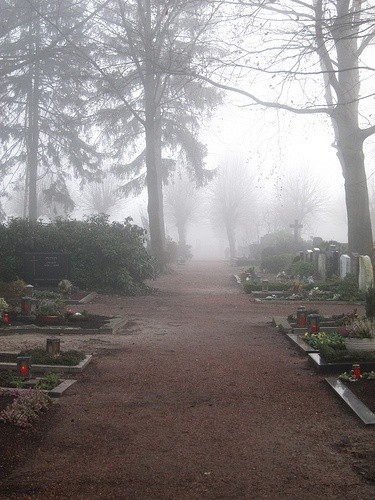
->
[68,312,91,329]
[34,299,60,324]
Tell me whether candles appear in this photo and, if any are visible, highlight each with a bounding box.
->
[353,367,361,378]
[19,364,27,378]
[2,313,9,321]
[22,303,30,313]
[311,323,317,333]
[299,315,305,326]
